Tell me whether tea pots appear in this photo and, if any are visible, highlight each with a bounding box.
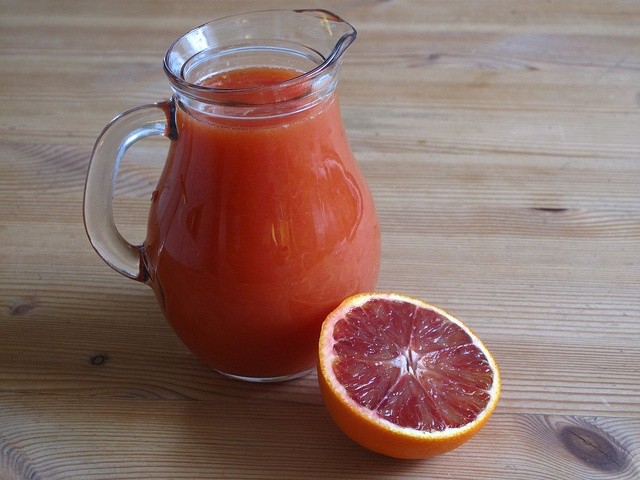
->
[83,8,382,384]
